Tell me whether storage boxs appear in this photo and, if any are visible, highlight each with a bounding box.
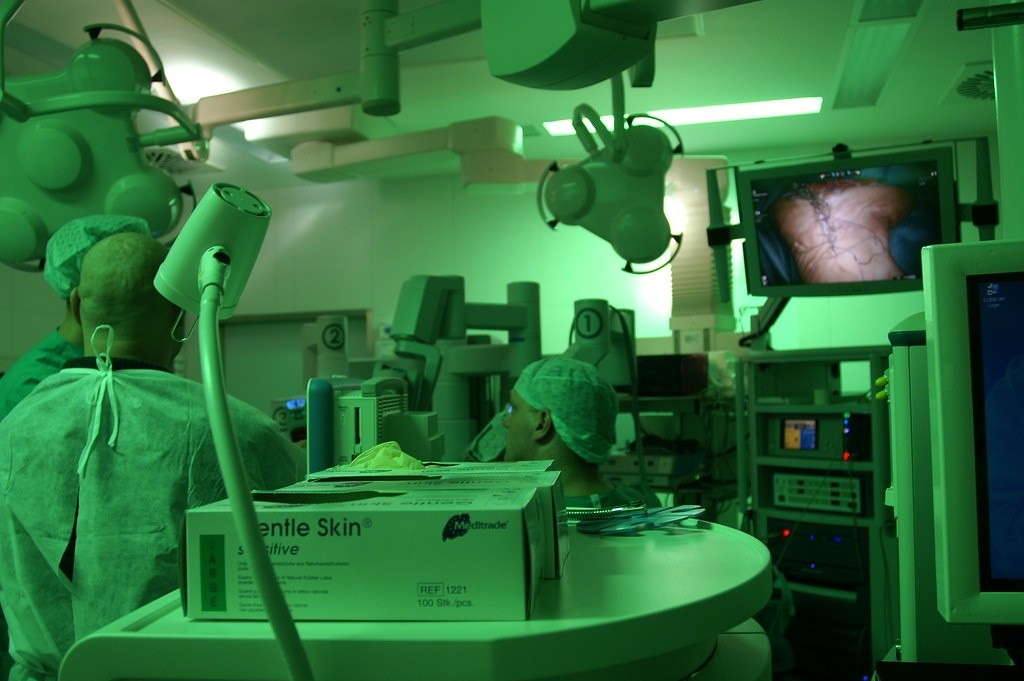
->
[176,460,570,624]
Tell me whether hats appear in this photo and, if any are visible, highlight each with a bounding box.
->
[513,356,618,463]
[44,214,152,299]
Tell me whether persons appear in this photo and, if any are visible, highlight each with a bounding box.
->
[774,185,915,284]
[0,213,306,681]
[502,355,632,509]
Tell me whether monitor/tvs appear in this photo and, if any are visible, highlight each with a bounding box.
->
[738,144,959,298]
[920,236,1023,626]
